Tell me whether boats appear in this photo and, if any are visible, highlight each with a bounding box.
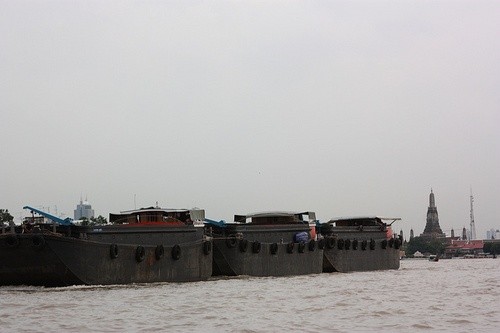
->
[207,209,326,274]
[19,205,216,283]
[429,248,496,261]
[315,216,403,272]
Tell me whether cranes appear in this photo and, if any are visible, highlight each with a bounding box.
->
[22,206,77,228]
